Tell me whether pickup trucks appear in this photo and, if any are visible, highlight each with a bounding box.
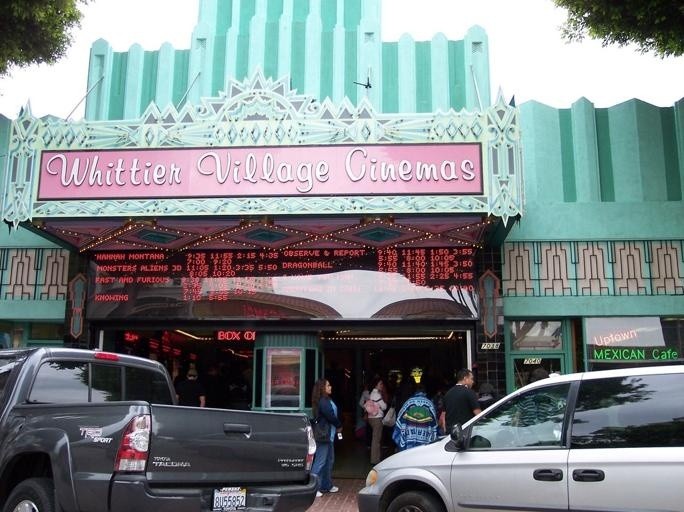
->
[0,345,318,512]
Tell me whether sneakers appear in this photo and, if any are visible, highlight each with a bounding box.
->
[315,491,323,497]
[328,485,340,493]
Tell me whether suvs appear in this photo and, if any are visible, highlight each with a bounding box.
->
[357,363,684,510]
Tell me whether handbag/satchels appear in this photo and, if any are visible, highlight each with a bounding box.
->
[361,398,380,417]
[309,415,332,443]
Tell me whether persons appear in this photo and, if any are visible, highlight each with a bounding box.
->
[175,369,207,408]
[511,368,559,448]
[310,378,343,497]
[440,368,481,437]
[355,372,444,466]
